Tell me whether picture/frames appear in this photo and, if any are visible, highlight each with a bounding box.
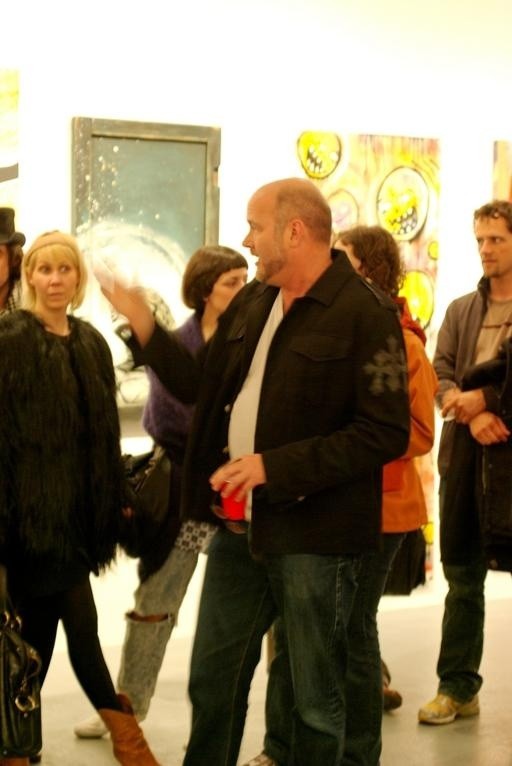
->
[64,116,223,423]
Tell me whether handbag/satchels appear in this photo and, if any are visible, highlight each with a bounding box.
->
[116,448,171,557]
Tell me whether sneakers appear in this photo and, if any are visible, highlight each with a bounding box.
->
[418,694,478,725]
[379,673,403,709]
[75,710,148,738]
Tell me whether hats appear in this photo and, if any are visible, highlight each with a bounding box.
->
[0,206,26,246]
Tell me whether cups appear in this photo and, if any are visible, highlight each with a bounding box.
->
[220,481,247,521]
[434,379,456,421]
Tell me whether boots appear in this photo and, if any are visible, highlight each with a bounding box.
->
[98,694,159,766]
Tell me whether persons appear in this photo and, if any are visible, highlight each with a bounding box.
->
[104,177,408,766]
[418,198,512,726]
[241,227,438,766]
[0,203,29,317]
[0,229,166,766]
[373,650,403,709]
[76,246,278,741]
